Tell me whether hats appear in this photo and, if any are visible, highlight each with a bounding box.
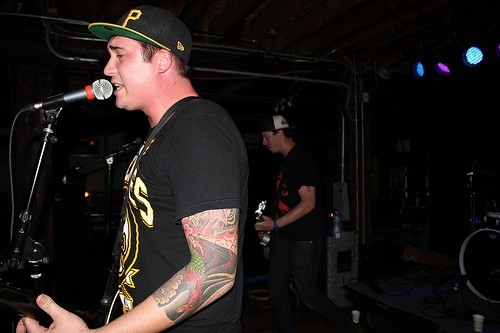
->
[258,115,298,131]
[88,5,192,64]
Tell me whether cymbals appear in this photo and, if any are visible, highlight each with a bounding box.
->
[456,159,495,175]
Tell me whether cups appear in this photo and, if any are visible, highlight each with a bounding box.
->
[352,310,360,324]
[472,314,485,332]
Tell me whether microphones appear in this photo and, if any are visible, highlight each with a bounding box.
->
[20,79,113,113]
[122,138,142,148]
[470,160,476,183]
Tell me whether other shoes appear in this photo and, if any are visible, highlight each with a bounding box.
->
[356,326,363,333]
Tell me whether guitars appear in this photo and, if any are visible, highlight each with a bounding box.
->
[0,283,120,333]
[255,200,272,246]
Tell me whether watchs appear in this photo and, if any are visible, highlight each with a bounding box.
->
[273,219,279,231]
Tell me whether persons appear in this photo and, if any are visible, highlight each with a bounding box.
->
[256,115,358,333]
[16,6,249,333]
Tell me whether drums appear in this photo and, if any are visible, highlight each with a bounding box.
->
[483,211,500,232]
[459,226,500,303]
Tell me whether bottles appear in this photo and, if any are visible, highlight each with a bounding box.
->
[332,211,342,239]
